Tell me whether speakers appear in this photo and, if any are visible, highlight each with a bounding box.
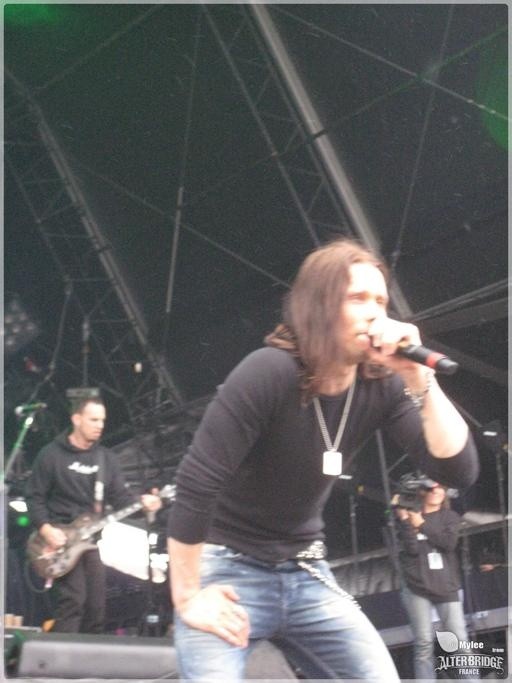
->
[6,631,178,680]
[242,640,298,678]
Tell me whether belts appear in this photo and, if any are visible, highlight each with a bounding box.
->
[273,540,328,565]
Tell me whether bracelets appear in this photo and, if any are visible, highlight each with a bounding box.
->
[403,368,435,409]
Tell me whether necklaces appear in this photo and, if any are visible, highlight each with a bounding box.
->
[313,377,358,477]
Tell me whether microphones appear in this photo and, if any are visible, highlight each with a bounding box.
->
[370,323,458,379]
[15,402,48,419]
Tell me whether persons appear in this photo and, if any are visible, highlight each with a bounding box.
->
[163,240,481,683]
[388,480,480,683]
[23,395,163,635]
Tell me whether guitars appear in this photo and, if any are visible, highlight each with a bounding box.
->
[27,482,177,577]
